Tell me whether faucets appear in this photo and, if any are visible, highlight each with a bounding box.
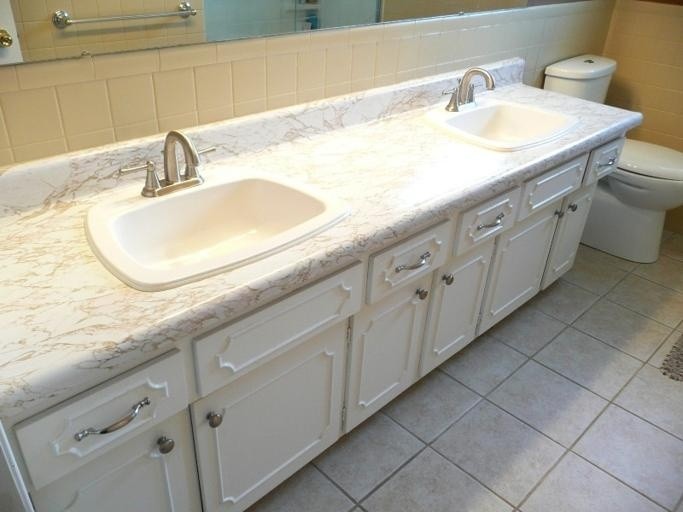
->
[163,129,199,184]
[457,68,498,105]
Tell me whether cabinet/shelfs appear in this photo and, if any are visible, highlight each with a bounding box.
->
[0,134,626,512]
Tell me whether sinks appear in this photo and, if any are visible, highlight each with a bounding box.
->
[425,99,579,151]
[84,172,353,293]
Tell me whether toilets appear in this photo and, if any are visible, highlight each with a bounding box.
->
[543,54,683,264]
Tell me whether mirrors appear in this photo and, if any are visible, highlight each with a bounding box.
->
[1,0,573,67]
